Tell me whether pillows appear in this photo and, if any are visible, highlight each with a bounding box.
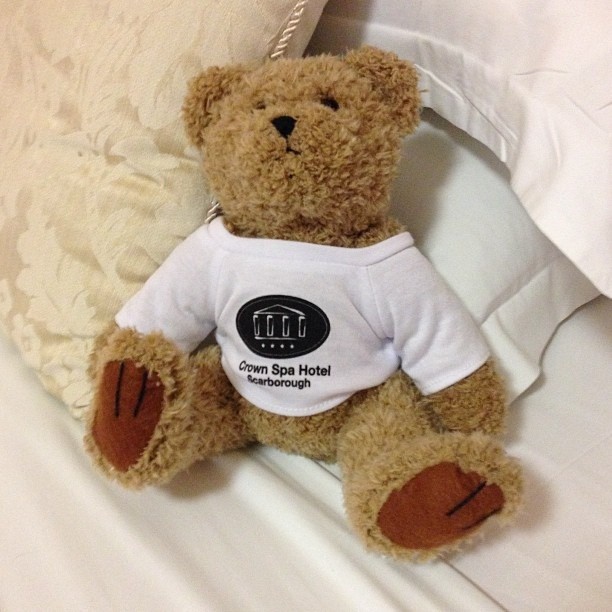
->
[1,0,330,428]
[396,103,606,404]
[307,1,608,299]
